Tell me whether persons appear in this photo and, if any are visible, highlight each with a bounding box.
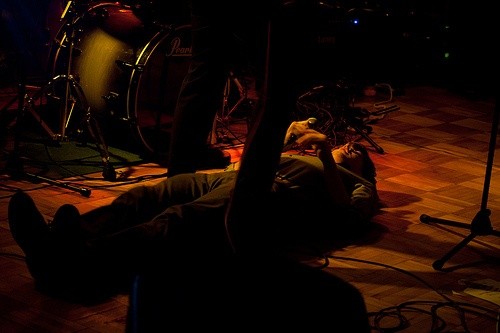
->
[162,0,321,263]
[6,117,380,305]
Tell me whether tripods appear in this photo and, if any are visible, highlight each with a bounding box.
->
[419,75,499,271]
[0,0,92,198]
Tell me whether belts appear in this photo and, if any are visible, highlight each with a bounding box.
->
[275,172,300,195]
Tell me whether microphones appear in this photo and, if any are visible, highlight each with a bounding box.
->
[285,117,318,145]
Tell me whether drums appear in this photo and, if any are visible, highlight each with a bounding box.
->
[48,1,212,156]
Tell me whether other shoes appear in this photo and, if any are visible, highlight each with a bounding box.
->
[49,204,103,301]
[8,191,62,294]
